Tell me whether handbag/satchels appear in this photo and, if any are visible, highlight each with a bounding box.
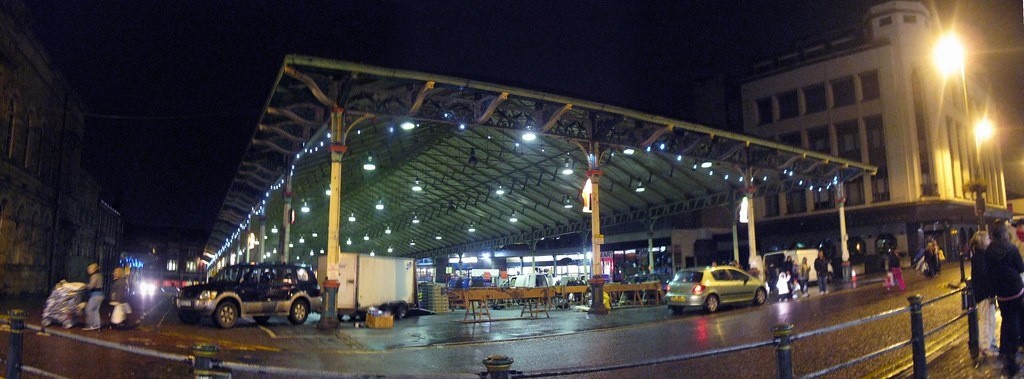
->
[884,272,895,287]
[110,302,132,324]
[939,250,945,261]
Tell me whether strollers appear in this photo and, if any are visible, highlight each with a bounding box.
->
[42,280,86,328]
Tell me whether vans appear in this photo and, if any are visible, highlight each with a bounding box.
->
[764,248,833,286]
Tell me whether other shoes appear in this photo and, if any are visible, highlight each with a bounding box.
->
[821,291,824,295]
[825,291,829,294]
[82,326,101,331]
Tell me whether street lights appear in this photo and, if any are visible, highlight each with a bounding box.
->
[930,33,989,232]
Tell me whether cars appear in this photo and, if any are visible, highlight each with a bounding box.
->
[665,265,770,312]
[502,275,550,304]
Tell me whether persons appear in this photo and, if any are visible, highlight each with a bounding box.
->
[109,267,129,331]
[924,240,942,278]
[886,245,906,292]
[776,256,811,302]
[711,260,738,266]
[82,262,105,331]
[814,251,829,295]
[968,218,1024,379]
[566,276,587,303]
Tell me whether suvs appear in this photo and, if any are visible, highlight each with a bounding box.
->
[177,264,323,329]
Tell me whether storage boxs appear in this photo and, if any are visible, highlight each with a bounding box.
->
[419,286,449,313]
[365,310,394,329]
